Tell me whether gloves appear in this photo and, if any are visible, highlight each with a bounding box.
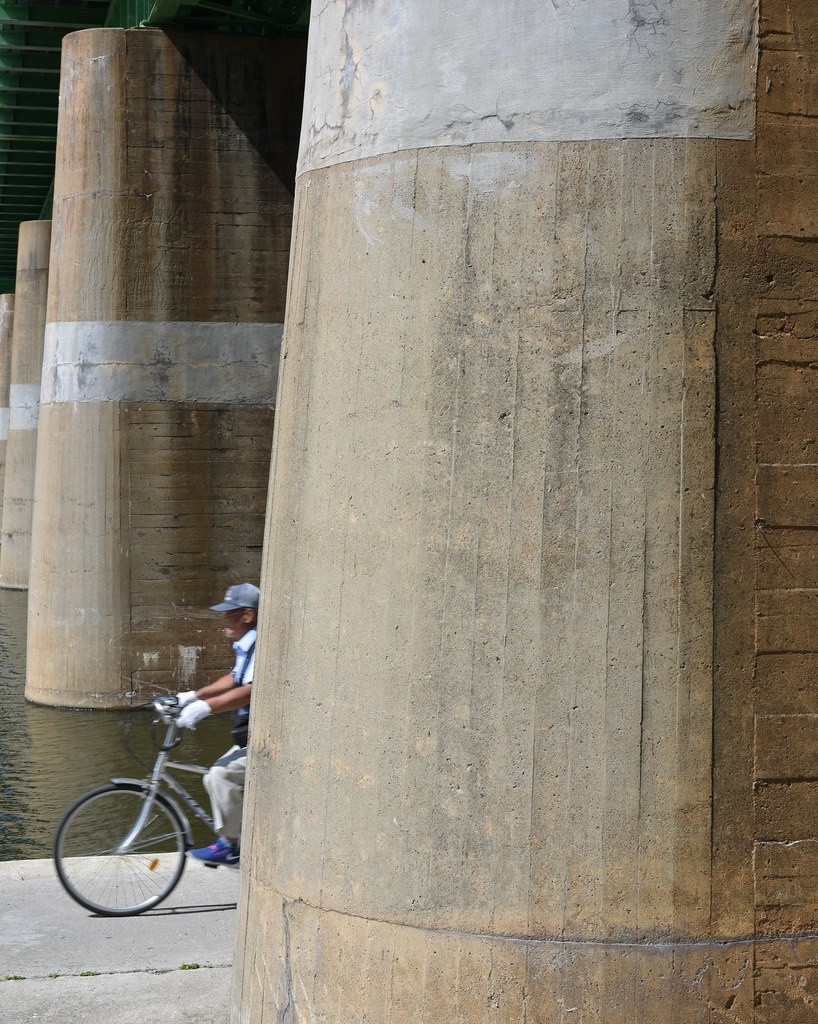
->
[176,699,211,731]
[173,690,198,709]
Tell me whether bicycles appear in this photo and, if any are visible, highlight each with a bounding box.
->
[53,694,227,917]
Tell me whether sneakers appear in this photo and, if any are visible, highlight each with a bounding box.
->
[183,837,240,870]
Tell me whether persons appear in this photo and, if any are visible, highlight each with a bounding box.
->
[155,581,262,870]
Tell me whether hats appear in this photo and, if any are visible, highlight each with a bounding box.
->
[209,584,260,615]
